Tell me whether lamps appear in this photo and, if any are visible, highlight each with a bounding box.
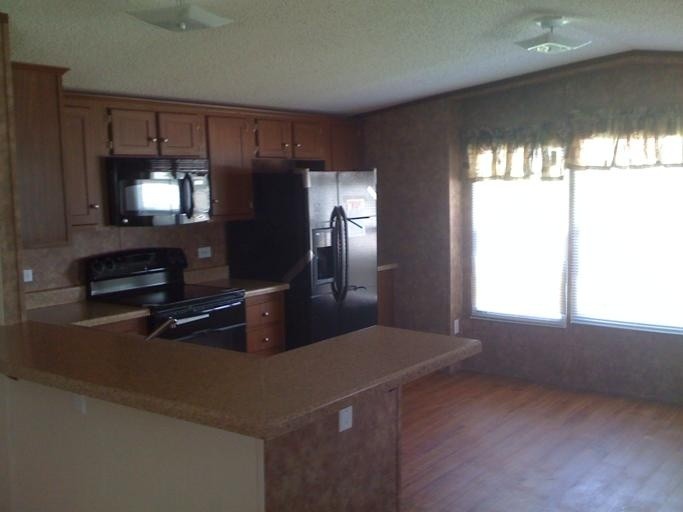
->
[512,15,591,55]
[124,2,238,34]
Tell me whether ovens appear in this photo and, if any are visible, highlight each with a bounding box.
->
[150,300,245,363]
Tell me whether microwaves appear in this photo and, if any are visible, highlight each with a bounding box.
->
[104,154,212,228]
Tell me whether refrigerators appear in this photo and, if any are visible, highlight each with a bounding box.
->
[255,167,379,349]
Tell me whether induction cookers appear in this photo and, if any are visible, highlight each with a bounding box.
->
[106,284,245,313]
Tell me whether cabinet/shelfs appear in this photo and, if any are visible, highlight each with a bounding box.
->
[108,94,210,156]
[208,101,256,221]
[59,86,109,229]
[242,288,287,355]
[254,106,329,159]
[7,60,70,250]
[88,313,149,338]
[295,115,364,175]
